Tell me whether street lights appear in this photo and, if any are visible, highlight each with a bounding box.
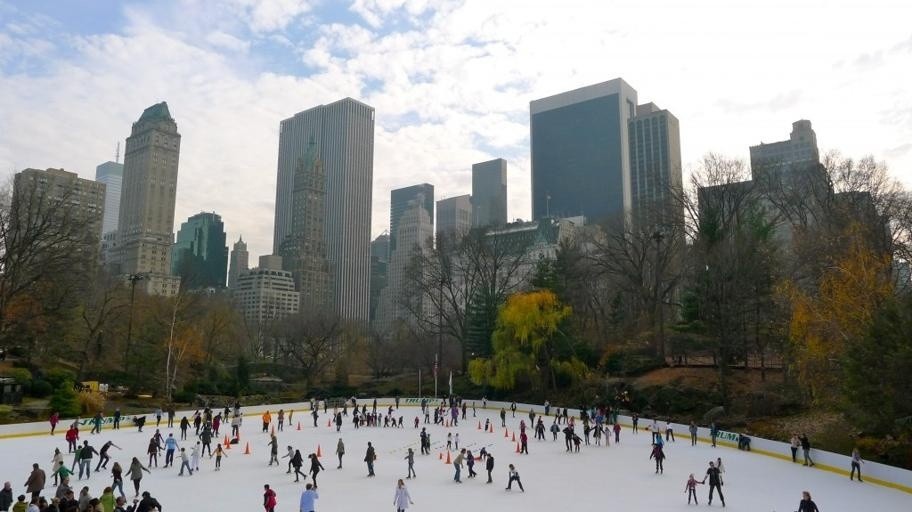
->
[126,271,148,361]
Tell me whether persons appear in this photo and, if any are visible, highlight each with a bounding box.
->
[1,384,726,512]
[795,491,819,512]
[738,434,751,451]
[791,434,801,462]
[798,433,815,466]
[851,443,864,481]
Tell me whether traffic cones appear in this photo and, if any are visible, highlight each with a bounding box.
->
[515,442,521,453]
[223,434,231,449]
[504,428,509,438]
[245,442,250,454]
[511,432,516,441]
[477,420,482,430]
[296,422,302,430]
[490,423,495,434]
[327,419,332,427]
[444,449,453,464]
[316,444,322,457]
